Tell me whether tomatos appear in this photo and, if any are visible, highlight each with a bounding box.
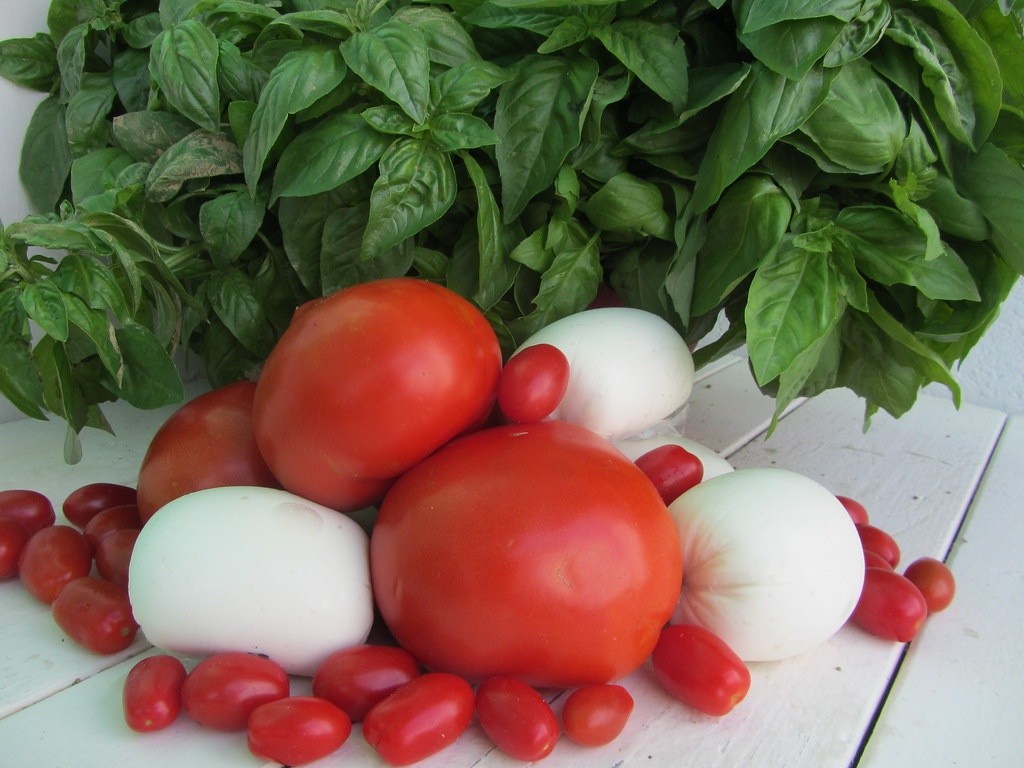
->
[0,273,954,768]
